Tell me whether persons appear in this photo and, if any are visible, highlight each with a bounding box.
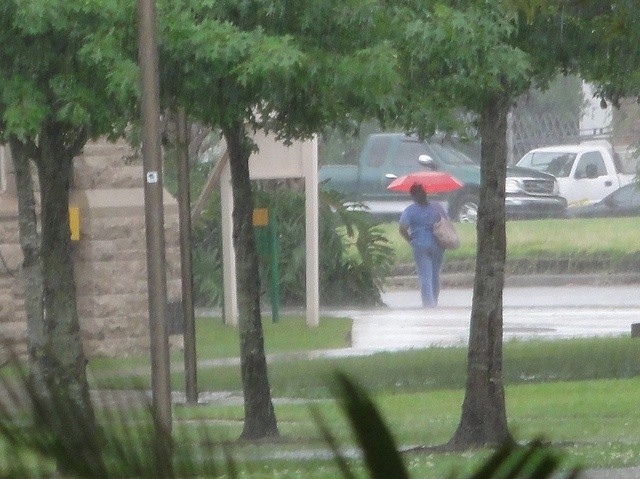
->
[399,183,449,308]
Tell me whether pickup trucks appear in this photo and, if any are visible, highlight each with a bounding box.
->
[317,132,568,225]
[514,141,640,219]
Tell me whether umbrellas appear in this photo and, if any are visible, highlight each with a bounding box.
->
[388,171,463,194]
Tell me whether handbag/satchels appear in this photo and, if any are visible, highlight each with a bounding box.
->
[434,213,459,249]
